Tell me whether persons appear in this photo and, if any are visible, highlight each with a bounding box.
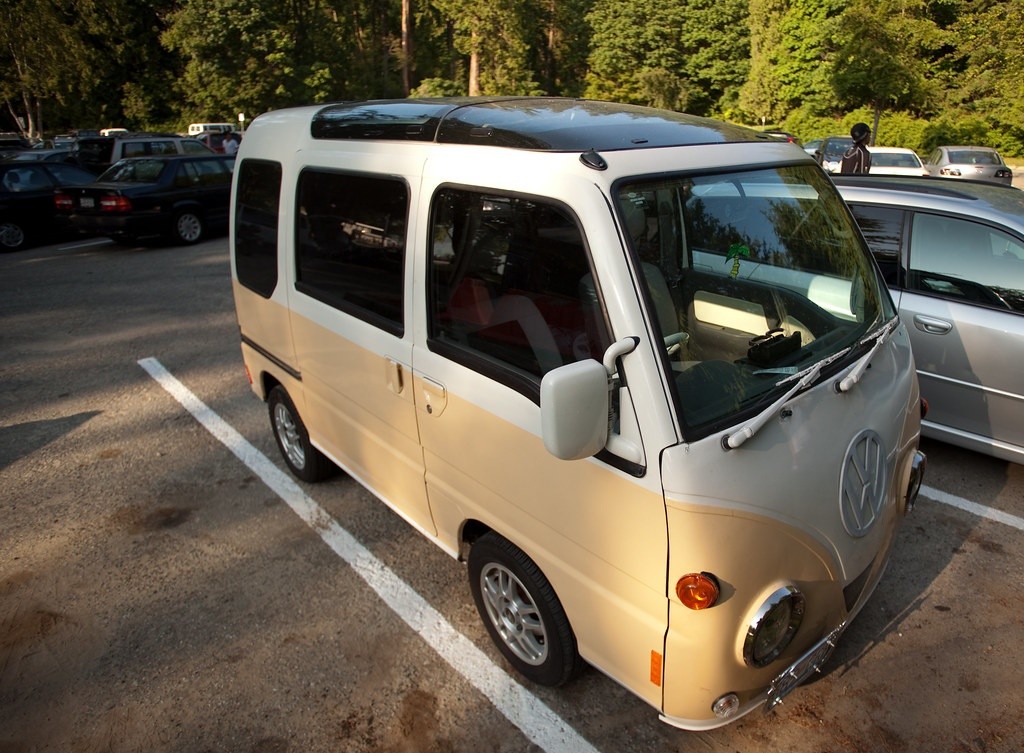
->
[222,131,238,152]
[29,131,43,146]
[841,123,872,174]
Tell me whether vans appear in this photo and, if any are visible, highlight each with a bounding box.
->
[225,93,928,731]
[816,134,856,175]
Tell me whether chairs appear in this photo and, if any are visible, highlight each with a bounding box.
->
[579,200,689,360]
[28,172,42,191]
[444,294,565,381]
[7,171,20,192]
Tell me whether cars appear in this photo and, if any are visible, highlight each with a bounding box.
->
[802,139,824,158]
[926,146,1013,186]
[616,171,1024,473]
[831,145,931,178]
[0,119,247,168]
[52,153,253,247]
[0,160,114,255]
